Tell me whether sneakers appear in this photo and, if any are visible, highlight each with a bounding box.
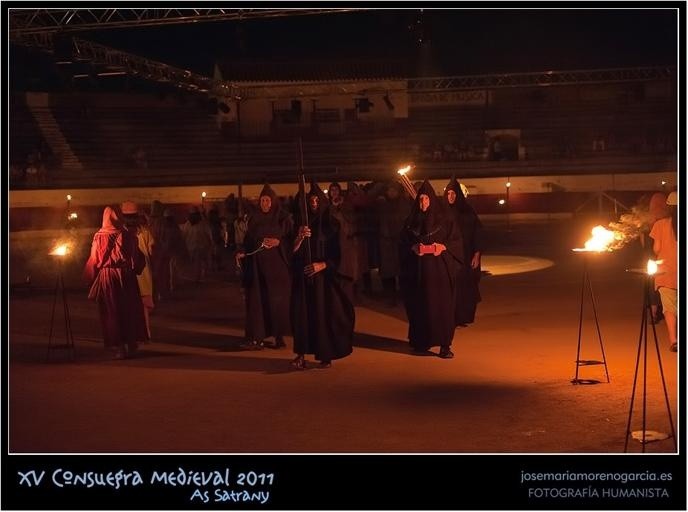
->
[409,339,454,358]
[112,341,138,361]
[670,342,677,352]
[233,336,287,351]
[363,287,397,308]
[649,316,658,324]
[289,353,331,370]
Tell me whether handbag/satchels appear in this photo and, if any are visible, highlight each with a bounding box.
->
[87,271,100,304]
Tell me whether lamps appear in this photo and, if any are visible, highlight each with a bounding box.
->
[52,35,128,78]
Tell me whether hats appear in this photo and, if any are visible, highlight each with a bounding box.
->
[665,192,677,206]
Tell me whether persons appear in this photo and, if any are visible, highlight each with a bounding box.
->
[144,201,173,308]
[280,180,356,372]
[634,187,677,354]
[234,181,294,352]
[120,200,156,340]
[396,179,466,358]
[440,178,483,329]
[83,204,148,360]
[158,173,424,311]
[482,138,519,162]
